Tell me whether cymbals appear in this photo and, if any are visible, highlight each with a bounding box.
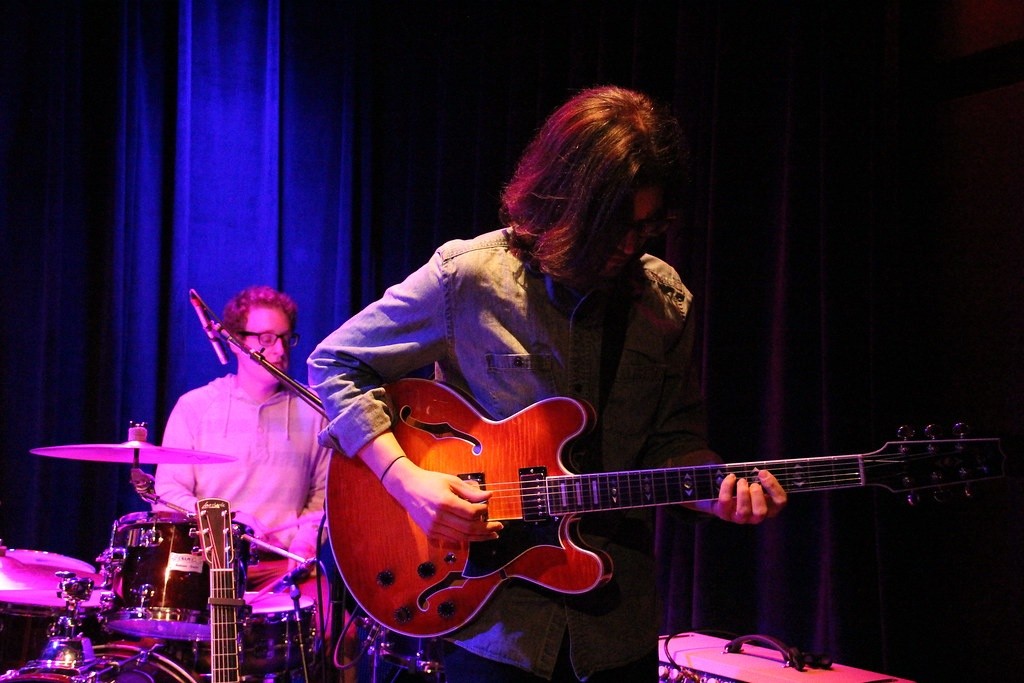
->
[0,556,107,590]
[29,440,239,464]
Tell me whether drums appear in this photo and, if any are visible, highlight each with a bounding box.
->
[0,549,114,683]
[165,590,317,683]
[98,510,254,641]
[354,616,445,683]
[0,644,196,683]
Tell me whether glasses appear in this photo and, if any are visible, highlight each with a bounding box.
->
[623,214,677,239]
[235,329,301,348]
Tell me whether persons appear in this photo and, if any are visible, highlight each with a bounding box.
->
[306,84,788,683]
[150,286,333,683]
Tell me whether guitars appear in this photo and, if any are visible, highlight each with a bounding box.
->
[324,377,1006,639]
[188,498,241,683]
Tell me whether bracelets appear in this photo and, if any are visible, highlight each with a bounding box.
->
[380,456,406,483]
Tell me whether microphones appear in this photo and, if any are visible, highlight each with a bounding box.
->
[189,290,229,364]
[273,558,319,594]
